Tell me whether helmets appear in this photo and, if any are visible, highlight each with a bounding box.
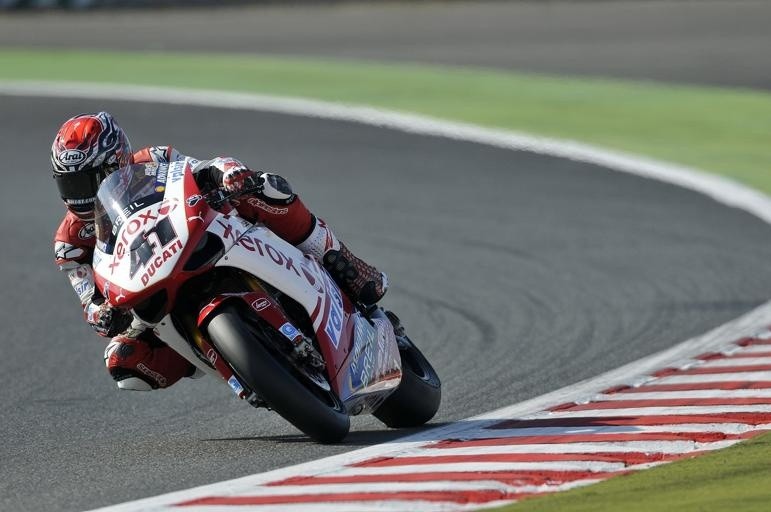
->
[50,110,133,222]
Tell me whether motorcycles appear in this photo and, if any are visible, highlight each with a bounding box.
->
[92,159,442,445]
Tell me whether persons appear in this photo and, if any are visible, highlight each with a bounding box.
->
[49,111,388,392]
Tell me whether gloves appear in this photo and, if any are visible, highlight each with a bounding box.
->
[222,164,258,207]
[95,296,134,338]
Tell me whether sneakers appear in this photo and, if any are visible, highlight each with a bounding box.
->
[296,215,389,305]
